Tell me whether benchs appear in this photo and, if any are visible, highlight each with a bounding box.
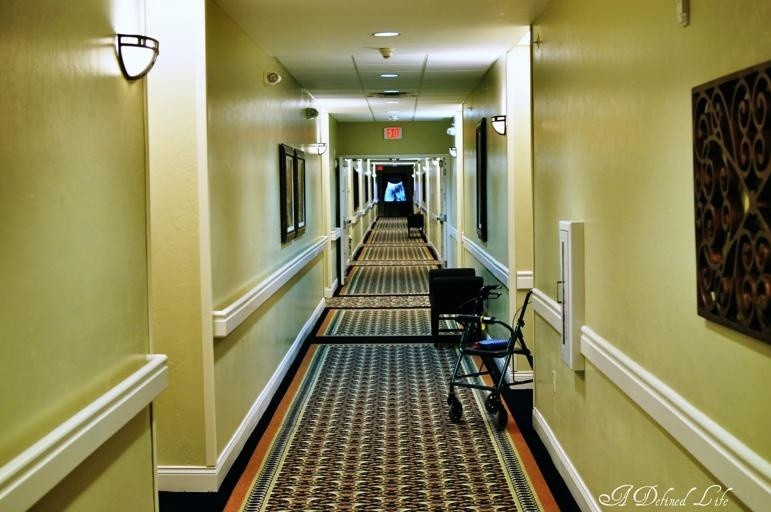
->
[427,267,484,348]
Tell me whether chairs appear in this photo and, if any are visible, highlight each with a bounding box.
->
[406,214,424,239]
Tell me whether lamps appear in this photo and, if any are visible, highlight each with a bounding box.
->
[115,32,159,82]
[318,143,327,154]
[448,147,457,157]
[492,114,507,137]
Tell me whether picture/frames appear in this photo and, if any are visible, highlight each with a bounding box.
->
[476,116,487,242]
[280,143,295,245]
[296,148,307,238]
[689,58,770,343]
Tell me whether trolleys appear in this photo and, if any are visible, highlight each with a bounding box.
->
[440,287,539,434]
